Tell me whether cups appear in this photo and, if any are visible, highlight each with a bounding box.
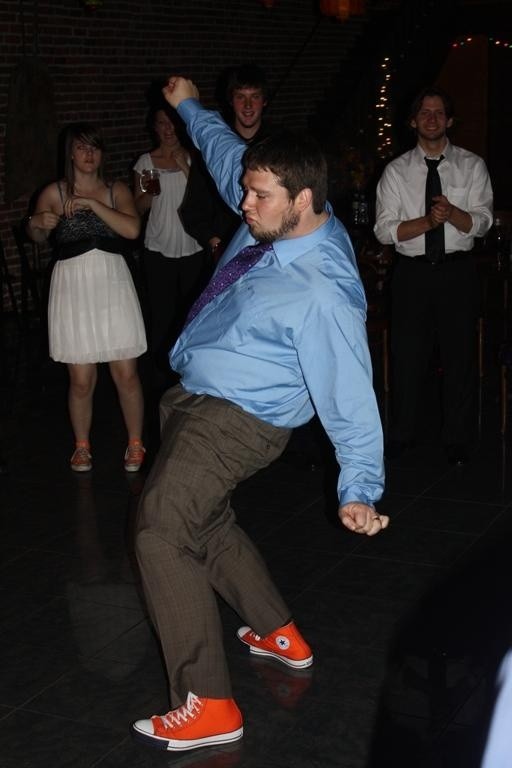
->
[138,169,161,198]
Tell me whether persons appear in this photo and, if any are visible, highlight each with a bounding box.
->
[132,103,211,359]
[375,90,494,479]
[130,72,389,756]
[25,124,149,473]
[175,68,280,270]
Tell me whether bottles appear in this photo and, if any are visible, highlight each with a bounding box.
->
[486,219,507,274]
[351,181,369,226]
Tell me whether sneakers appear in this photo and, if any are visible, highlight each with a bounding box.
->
[71,440,93,471]
[124,438,145,471]
[132,692,243,751]
[236,619,314,669]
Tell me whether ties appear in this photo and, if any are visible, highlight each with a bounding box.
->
[183,240,274,330]
[424,156,447,277]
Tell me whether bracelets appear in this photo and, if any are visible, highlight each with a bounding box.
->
[28,215,33,229]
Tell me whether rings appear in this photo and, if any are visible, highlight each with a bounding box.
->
[371,516,380,521]
